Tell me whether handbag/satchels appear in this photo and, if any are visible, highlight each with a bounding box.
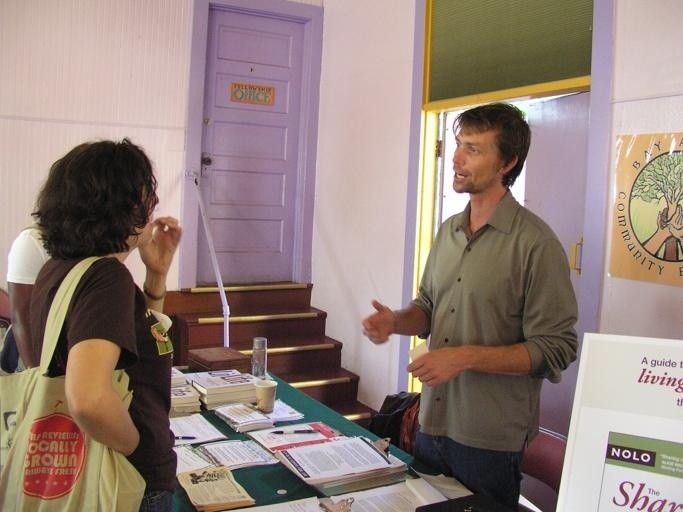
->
[0,366,148,511]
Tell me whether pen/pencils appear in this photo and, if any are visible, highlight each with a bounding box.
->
[175,436,196,439]
[271,430,320,434]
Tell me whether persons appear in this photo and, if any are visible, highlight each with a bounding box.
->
[33,138,181,511]
[363,101,576,511]
[4,219,53,370]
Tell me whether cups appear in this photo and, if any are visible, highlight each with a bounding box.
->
[255,380,277,412]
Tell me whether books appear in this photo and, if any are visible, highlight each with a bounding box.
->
[168,367,501,511]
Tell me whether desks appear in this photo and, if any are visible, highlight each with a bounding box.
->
[158,368,475,512]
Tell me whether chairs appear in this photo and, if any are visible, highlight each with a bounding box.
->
[517,425,568,512]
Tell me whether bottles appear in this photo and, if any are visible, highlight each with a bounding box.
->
[252,337,267,378]
